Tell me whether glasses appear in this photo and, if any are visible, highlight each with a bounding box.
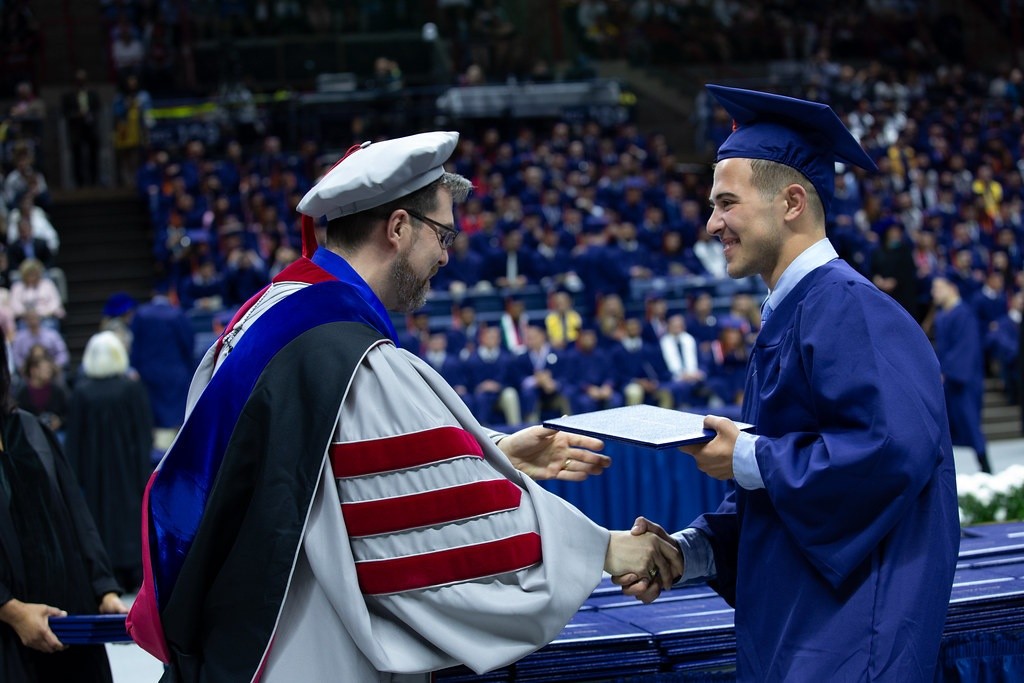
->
[388,210,458,248]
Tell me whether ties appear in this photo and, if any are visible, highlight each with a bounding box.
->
[760,299,773,330]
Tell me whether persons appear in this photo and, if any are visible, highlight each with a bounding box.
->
[1,2,1024,429]
[612,82,959,683]
[0,326,129,683]
[926,260,995,479]
[130,133,683,683]
[59,332,154,595]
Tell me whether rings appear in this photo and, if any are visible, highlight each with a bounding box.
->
[563,459,574,471]
[649,565,658,579]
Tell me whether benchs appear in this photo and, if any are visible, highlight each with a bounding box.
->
[0,1,1024,483]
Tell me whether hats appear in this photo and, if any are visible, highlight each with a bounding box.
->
[704,83,879,219]
[295,131,461,252]
